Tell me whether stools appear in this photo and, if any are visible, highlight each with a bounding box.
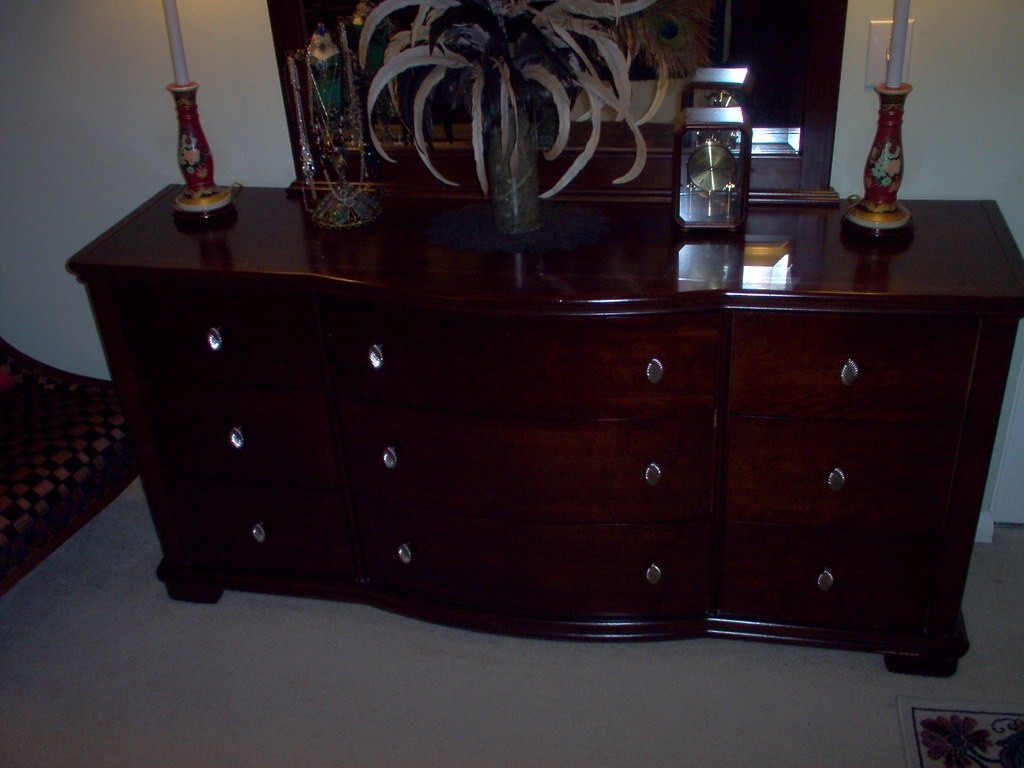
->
[0,339,140,601]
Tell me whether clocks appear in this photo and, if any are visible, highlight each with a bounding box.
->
[668,105,753,234]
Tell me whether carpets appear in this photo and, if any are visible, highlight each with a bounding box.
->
[897,694,1024,768]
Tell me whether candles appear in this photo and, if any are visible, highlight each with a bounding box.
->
[883,0,911,88]
[161,0,190,87]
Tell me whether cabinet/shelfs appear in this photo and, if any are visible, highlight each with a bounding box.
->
[64,179,1024,679]
